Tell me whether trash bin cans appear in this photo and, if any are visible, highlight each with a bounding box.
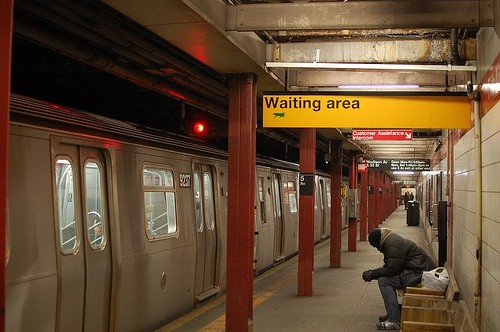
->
[407,200,419,226]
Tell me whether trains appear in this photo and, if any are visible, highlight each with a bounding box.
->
[0,91,368,332]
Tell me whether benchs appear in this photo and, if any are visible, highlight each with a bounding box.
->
[400,262,479,332]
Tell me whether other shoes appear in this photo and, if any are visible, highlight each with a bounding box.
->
[379,315,388,320]
[377,321,400,330]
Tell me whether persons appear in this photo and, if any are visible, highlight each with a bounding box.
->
[362,227,431,330]
[94,218,103,245]
[401,191,414,210]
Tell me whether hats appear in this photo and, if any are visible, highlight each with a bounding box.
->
[368,229,381,247]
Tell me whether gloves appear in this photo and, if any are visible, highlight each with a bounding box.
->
[362,269,374,282]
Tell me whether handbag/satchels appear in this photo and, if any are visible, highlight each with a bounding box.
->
[421,266,449,289]
